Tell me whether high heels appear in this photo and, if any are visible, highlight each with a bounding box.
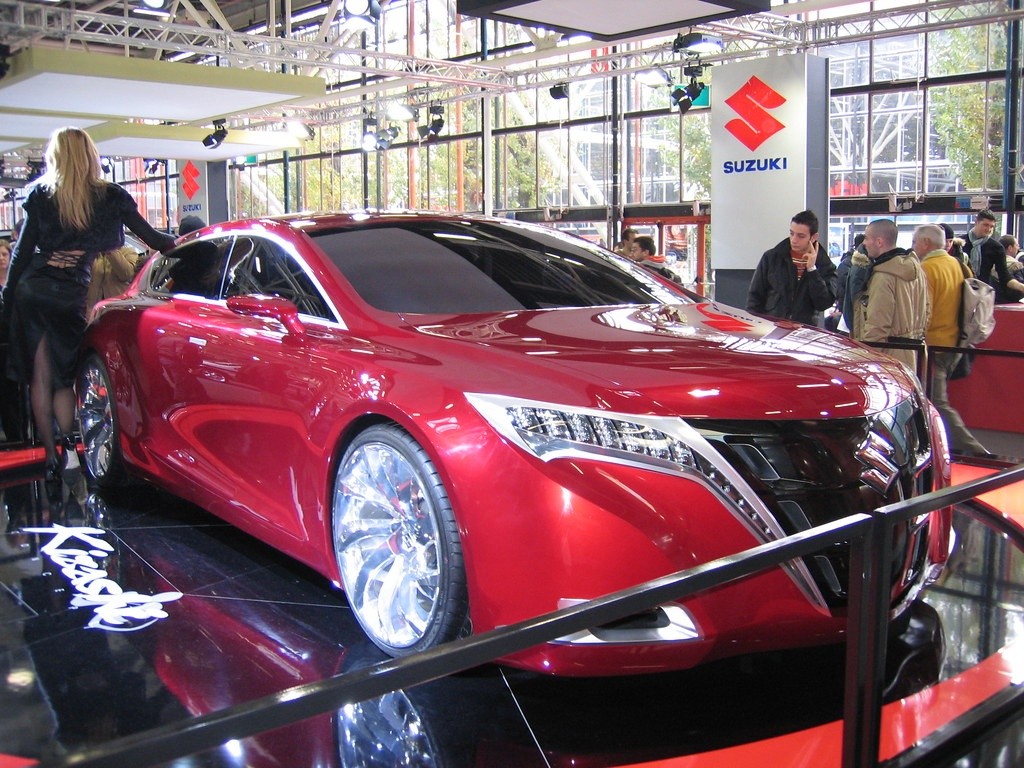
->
[58,433,82,488]
[42,457,62,483]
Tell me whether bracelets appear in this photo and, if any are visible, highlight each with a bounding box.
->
[806,266,816,271]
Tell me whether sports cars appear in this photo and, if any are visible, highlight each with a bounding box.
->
[73,212,955,677]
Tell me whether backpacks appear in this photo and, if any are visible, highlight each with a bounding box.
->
[956,255,998,348]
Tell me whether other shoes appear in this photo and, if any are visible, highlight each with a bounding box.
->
[979,450,998,459]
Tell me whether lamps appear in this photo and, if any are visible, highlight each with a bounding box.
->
[100,156,115,173]
[344,0,382,25]
[202,118,229,149]
[232,156,245,172]
[632,66,675,89]
[143,159,165,174]
[363,127,399,152]
[4,190,18,201]
[26,161,47,182]
[549,82,569,99]
[669,31,723,114]
[133,0,177,18]
[417,102,444,144]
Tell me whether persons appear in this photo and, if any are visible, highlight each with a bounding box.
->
[631,236,684,285]
[954,209,1024,303]
[7,218,40,253]
[991,233,1024,304]
[842,239,875,339]
[745,209,838,328]
[938,222,975,280]
[179,214,205,239]
[836,234,865,313]
[83,238,137,323]
[910,223,997,458]
[861,218,930,376]
[614,228,641,263]
[0,239,14,304]
[0,128,181,484]
